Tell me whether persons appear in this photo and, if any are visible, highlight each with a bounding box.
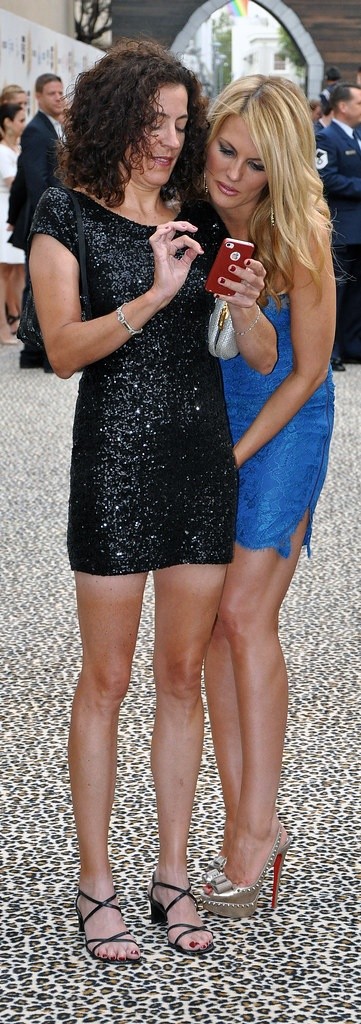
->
[23,42,280,962]
[1,73,361,373]
[185,69,340,921]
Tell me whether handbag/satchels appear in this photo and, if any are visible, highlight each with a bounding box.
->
[16,187,91,346]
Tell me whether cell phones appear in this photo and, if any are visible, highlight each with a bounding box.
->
[205,237,255,296]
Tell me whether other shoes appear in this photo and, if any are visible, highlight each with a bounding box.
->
[0,334,21,345]
[6,303,20,334]
[20,350,40,369]
[331,360,345,371]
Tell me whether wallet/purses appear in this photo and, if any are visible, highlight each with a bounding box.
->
[208,296,240,360]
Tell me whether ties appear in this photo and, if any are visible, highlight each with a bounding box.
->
[352,131,361,150]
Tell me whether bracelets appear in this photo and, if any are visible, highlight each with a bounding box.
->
[116,301,144,337]
[230,304,262,336]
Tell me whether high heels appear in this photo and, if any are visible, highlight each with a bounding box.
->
[73,887,140,963]
[201,824,293,917]
[147,872,216,954]
